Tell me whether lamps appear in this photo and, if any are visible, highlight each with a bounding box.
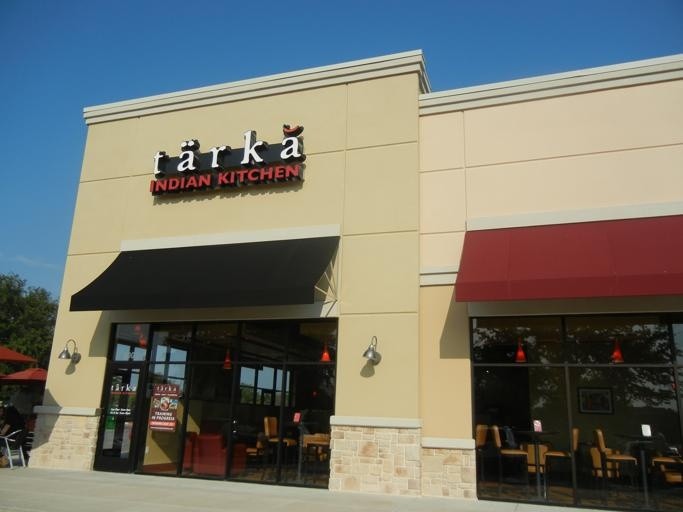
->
[58,337,82,366]
[514,335,526,364]
[223,349,232,371]
[609,337,622,364]
[363,333,379,364]
[319,334,331,364]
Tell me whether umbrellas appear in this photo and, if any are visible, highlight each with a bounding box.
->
[0,346,38,362]
[0,368,47,405]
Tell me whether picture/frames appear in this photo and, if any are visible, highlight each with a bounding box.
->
[577,385,616,417]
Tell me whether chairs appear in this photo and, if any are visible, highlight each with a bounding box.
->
[0,428,28,471]
[193,414,330,489]
[472,425,682,508]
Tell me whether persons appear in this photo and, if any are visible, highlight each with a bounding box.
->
[0,407,25,467]
[0,406,5,430]
